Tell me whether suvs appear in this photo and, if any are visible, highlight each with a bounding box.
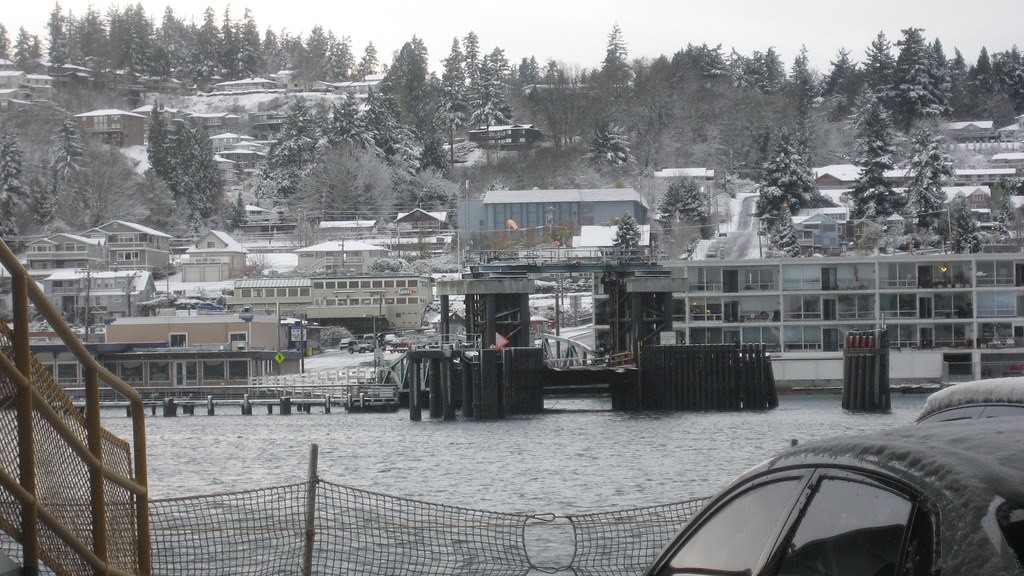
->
[198,303,224,312]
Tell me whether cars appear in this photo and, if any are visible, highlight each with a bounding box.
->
[339,330,437,354]
[707,248,717,257]
[718,231,727,236]
[639,380,1024,576]
